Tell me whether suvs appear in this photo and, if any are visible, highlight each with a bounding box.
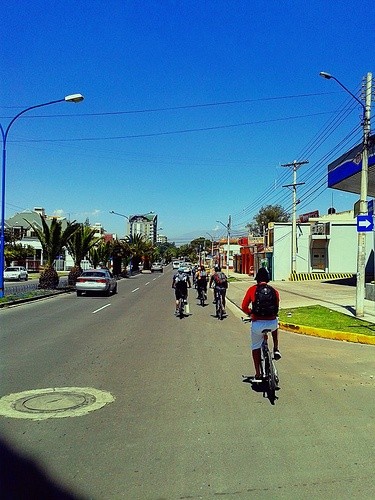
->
[151,262,164,273]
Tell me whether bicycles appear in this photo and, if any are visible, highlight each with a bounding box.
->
[173,286,190,319]
[195,280,208,306]
[210,286,225,321]
[242,315,279,404]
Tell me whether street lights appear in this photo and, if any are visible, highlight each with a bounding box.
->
[206,232,214,255]
[216,220,231,277]
[319,71,375,318]
[0,92,84,298]
[109,211,164,271]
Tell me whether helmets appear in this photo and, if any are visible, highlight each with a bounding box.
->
[178,268,184,272]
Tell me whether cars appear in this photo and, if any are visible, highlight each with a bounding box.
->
[173,260,204,276]
[4,266,28,281]
[75,268,118,297]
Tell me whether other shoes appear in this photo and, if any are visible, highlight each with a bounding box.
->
[255,374,262,380]
[274,350,282,360]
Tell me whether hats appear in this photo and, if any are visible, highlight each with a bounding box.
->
[254,267,270,281]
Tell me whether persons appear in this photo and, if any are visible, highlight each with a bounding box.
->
[241,267,282,379]
[192,265,208,300]
[172,268,191,313]
[209,266,228,308]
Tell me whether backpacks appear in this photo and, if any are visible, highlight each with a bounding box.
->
[251,284,278,318]
[175,273,187,287]
[214,272,228,288]
